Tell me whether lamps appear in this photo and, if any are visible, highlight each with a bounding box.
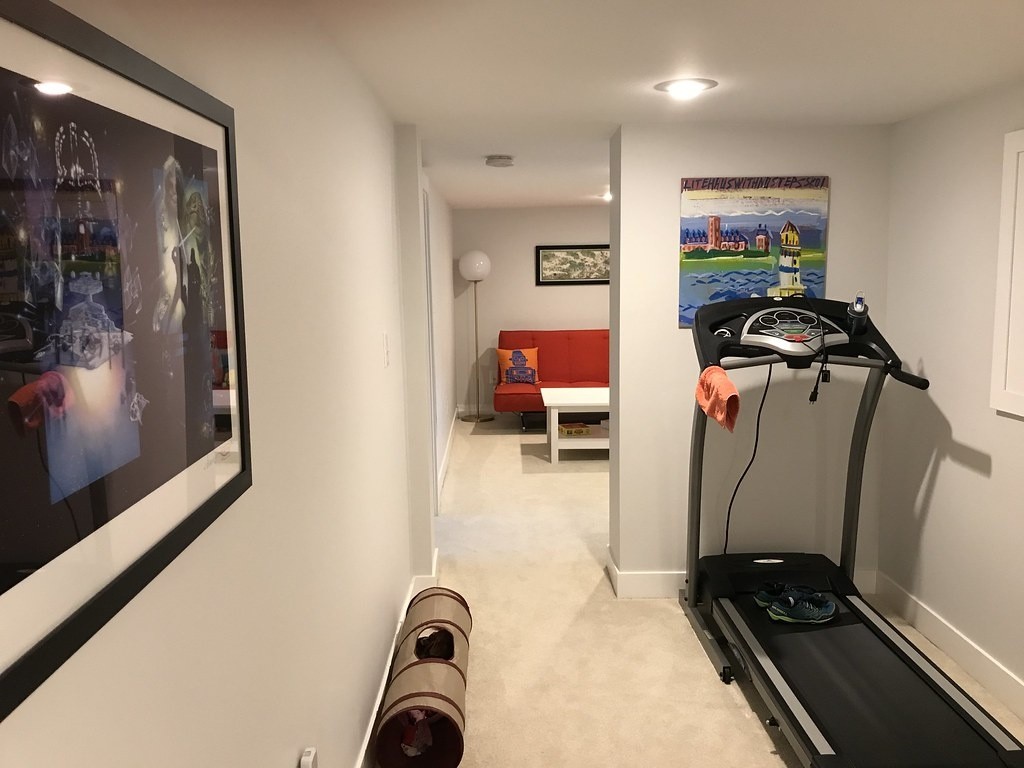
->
[457,247,497,422]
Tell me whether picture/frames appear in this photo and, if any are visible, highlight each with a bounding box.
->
[0,0,256,721]
[675,175,834,347]
[533,243,611,287]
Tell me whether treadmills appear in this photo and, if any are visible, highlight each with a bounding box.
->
[678,294,1023,768]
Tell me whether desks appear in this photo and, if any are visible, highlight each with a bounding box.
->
[536,385,611,467]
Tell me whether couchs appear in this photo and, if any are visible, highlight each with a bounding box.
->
[491,329,611,429]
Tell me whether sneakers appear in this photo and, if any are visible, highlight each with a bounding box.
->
[752,578,841,625]
